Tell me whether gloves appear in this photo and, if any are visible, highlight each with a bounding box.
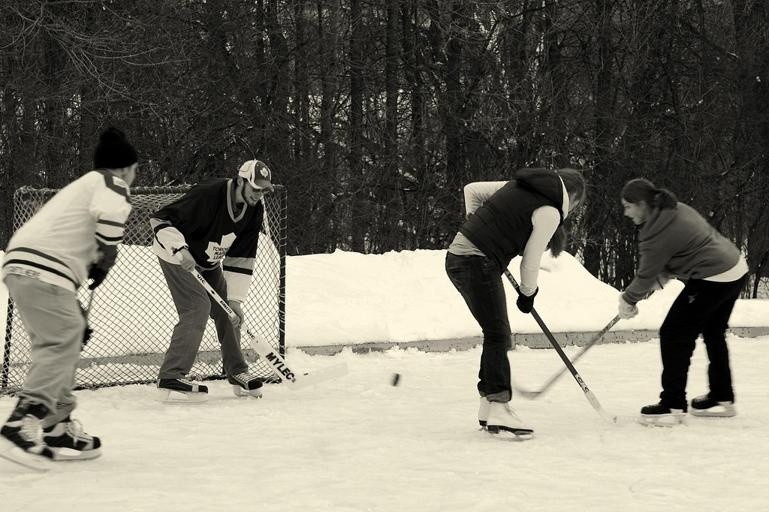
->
[88,267,106,290]
[517,287,538,313]
[619,295,638,319]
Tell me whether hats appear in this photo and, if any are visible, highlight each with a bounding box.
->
[94,127,138,170]
[238,160,274,193]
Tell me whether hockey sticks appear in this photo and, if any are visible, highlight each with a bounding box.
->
[176,248,352,389]
[508,271,691,425]
[514,312,620,398]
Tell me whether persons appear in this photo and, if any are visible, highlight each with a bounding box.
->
[445,165,585,436]
[617,176,751,417]
[148,157,277,394]
[0,126,140,459]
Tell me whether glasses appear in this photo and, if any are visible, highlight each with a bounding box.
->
[252,188,271,192]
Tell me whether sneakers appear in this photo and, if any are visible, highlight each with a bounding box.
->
[487,402,533,435]
[1,404,52,458]
[43,416,100,450]
[642,398,688,414]
[692,393,734,409]
[228,372,262,390]
[478,396,489,426]
[157,378,208,393]
